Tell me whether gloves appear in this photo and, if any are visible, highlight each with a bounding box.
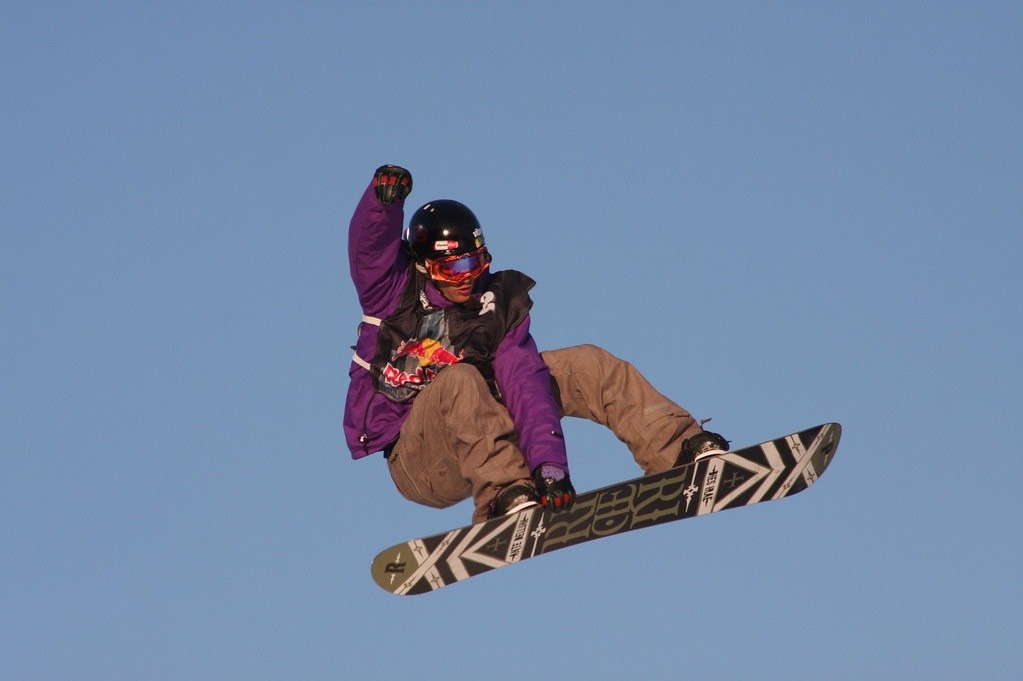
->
[374,164,414,203]
[528,465,576,512]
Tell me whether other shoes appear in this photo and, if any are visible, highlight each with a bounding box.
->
[680,431,727,463]
[491,486,538,517]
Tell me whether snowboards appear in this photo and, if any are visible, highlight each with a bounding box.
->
[369,421,842,596]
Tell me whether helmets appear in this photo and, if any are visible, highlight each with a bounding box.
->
[409,199,484,263]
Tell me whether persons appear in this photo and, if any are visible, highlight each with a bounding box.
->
[343,165,730,525]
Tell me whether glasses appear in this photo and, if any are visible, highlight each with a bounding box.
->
[430,247,491,284]
